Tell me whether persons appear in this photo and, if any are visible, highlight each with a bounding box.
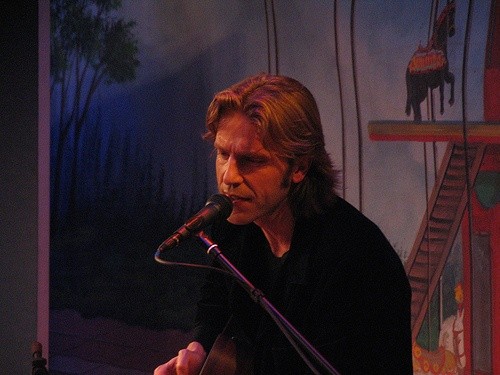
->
[152,72,415,375]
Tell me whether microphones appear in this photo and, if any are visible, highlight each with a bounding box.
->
[156,192,233,256]
